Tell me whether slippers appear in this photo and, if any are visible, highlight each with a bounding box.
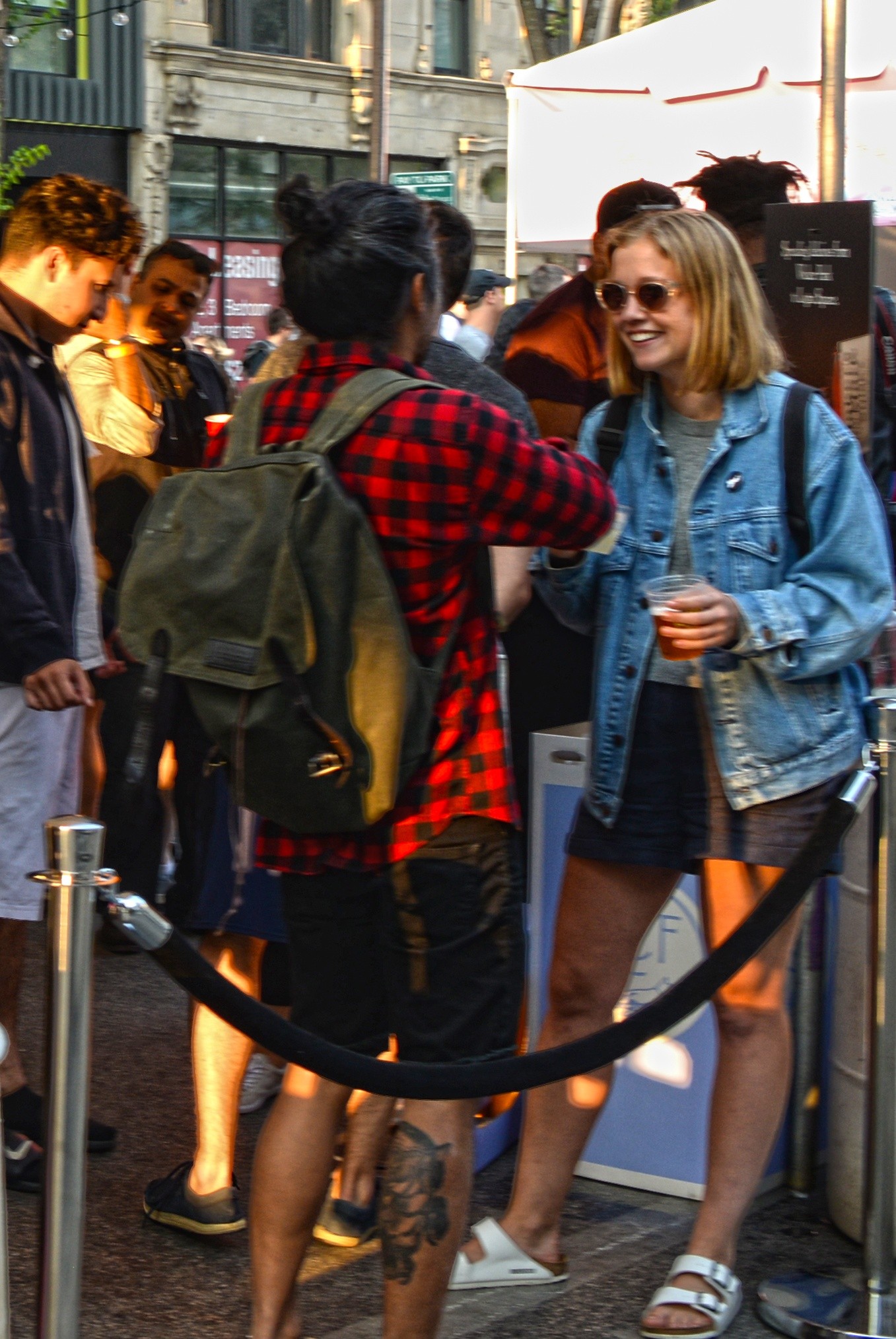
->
[444,1218,573,1294]
[635,1259,743,1339]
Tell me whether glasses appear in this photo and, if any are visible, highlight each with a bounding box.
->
[151,239,220,276]
[595,281,688,311]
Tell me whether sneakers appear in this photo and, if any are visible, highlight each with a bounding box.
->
[3,1087,117,1148]
[240,1058,288,1111]
[6,1130,51,1195]
[309,1179,389,1248]
[143,1160,256,1235]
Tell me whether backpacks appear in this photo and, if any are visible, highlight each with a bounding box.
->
[119,364,475,838]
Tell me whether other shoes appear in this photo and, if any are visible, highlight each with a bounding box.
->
[90,917,137,953]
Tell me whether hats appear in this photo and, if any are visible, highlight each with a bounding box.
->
[464,270,511,306]
[596,178,682,232]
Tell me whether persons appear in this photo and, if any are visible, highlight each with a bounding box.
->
[1,149,896,1339]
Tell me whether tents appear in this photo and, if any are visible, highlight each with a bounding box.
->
[502,0,896,307]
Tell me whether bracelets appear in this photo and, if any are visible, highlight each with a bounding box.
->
[103,342,138,359]
[102,334,128,345]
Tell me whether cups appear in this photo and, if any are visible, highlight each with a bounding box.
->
[205,414,233,436]
[642,574,709,660]
